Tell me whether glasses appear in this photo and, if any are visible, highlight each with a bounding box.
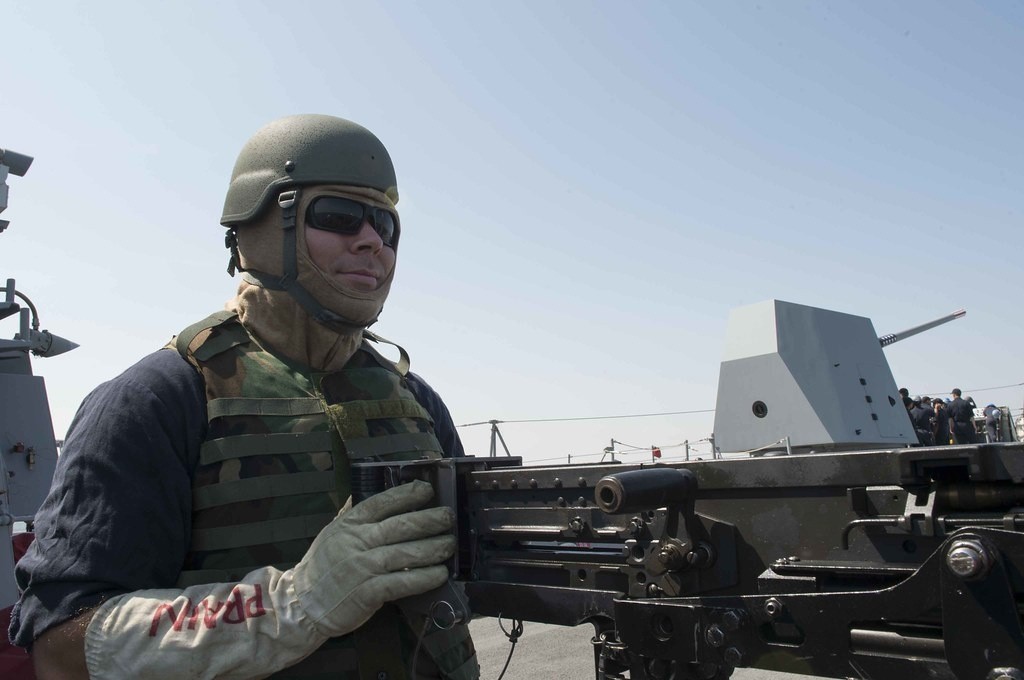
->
[304,195,400,251]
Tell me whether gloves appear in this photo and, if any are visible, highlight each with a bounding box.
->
[84,481,459,680]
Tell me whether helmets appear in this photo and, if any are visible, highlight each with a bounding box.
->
[220,114,399,224]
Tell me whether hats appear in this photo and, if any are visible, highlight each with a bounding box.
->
[923,396,933,402]
[949,388,961,394]
[912,395,922,401]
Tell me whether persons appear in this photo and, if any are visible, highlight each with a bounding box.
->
[899,387,977,446]
[9,114,481,680]
[984,404,1000,443]
